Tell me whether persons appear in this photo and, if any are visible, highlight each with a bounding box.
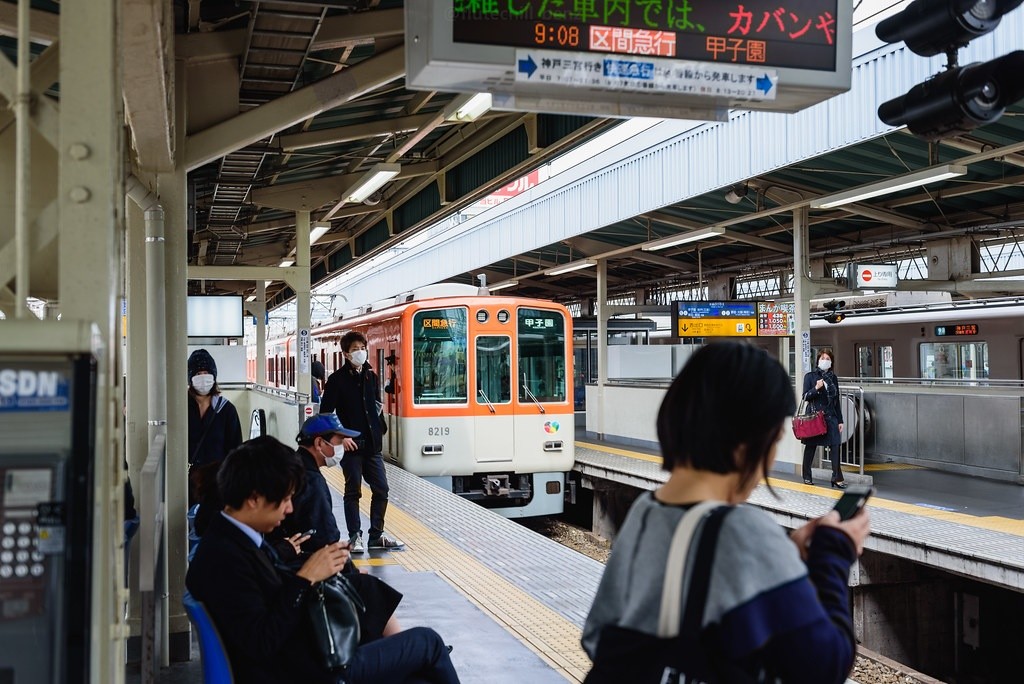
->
[492,374,509,400]
[320,332,406,551]
[581,341,869,684]
[188,349,242,508]
[802,347,848,488]
[311,361,324,403]
[385,355,395,394]
[185,414,460,684]
[423,356,439,390]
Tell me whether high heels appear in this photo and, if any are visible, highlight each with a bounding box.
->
[831,481,846,489]
[804,478,814,485]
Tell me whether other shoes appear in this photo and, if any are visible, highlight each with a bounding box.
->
[445,645,453,653]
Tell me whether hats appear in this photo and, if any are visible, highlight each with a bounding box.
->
[187,349,217,384]
[299,413,361,437]
[384,355,395,366]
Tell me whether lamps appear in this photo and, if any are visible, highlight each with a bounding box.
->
[341,163,401,204]
[278,258,295,268]
[544,260,598,276]
[443,92,493,123]
[725,184,745,204]
[810,165,967,209]
[291,222,331,254]
[245,280,272,301]
[643,226,725,252]
[486,280,518,292]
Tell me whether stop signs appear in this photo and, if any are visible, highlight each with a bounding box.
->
[862,270,872,281]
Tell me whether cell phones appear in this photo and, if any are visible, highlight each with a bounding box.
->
[344,530,363,549]
[805,486,872,547]
[295,529,316,542]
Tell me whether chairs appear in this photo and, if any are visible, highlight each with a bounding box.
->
[180,503,238,684]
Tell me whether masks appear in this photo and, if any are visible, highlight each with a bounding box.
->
[320,441,344,468]
[191,374,215,396]
[818,360,831,370]
[347,350,367,366]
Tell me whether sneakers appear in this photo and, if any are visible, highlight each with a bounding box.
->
[347,532,364,552]
[367,534,405,549]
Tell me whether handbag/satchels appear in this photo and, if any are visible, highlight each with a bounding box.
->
[585,502,805,683]
[792,392,827,439]
[297,572,365,671]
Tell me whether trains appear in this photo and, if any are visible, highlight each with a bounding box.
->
[247,297,576,519]
[577,301,1023,483]
